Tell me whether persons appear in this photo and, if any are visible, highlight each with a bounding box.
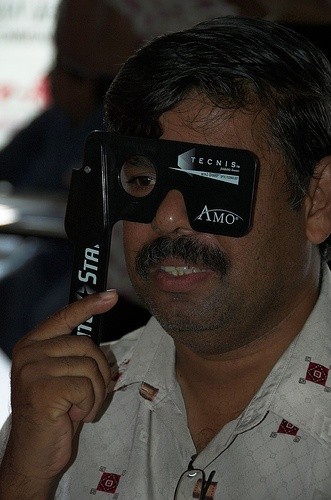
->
[2,1,331,500]
